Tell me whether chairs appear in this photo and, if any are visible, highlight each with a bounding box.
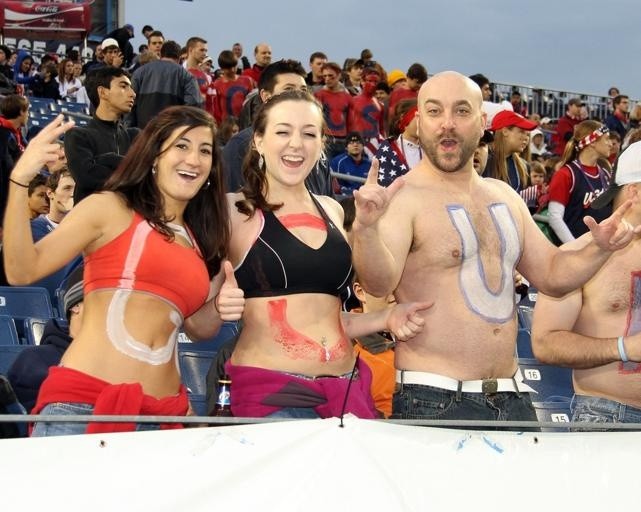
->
[1,284,574,432]
[25,93,94,177]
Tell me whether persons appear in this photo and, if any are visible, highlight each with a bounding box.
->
[1,23,640,437]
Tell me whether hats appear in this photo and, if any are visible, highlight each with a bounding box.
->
[387,70,407,88]
[101,38,119,50]
[569,99,585,106]
[491,110,537,130]
[615,141,641,187]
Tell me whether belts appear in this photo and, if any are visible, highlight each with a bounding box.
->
[396,366,538,393]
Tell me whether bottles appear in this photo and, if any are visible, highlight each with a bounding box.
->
[207,374,235,426]
